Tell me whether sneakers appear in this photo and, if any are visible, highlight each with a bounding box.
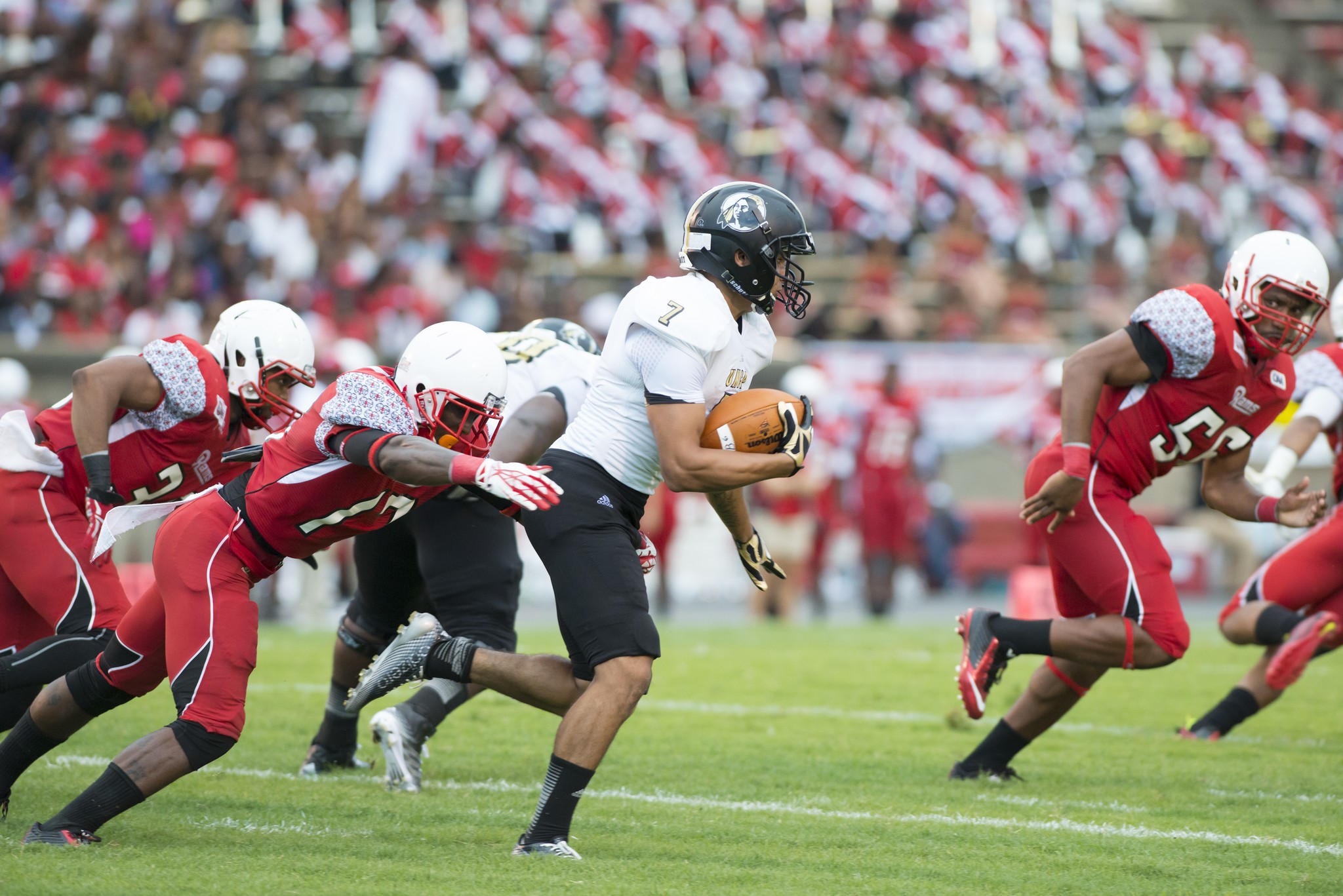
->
[366,703,436,789]
[298,743,366,777]
[1173,717,1223,740]
[343,610,453,719]
[20,821,99,848]
[953,606,1018,720]
[1266,612,1341,691]
[510,833,583,859]
[946,761,1027,784]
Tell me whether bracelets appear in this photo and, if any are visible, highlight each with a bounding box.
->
[1254,496,1278,524]
[1062,441,1091,481]
[449,453,490,485]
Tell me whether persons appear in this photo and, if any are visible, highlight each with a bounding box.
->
[756,364,837,621]
[300,319,603,778]
[0,0,1343,343]
[1177,277,1343,743]
[946,230,1329,778]
[1,321,564,844]
[0,300,316,732]
[345,180,815,859]
[865,366,915,618]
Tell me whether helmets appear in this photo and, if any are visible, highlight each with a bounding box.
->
[678,182,817,316]
[1217,230,1331,320]
[395,320,509,436]
[203,298,316,403]
[519,316,606,357]
[1329,276,1342,340]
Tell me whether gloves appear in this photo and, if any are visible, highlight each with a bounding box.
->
[634,528,657,574]
[84,485,122,569]
[735,525,789,591]
[472,457,565,512]
[778,394,812,478]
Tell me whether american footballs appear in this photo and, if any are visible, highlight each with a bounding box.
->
[693,388,807,465]
